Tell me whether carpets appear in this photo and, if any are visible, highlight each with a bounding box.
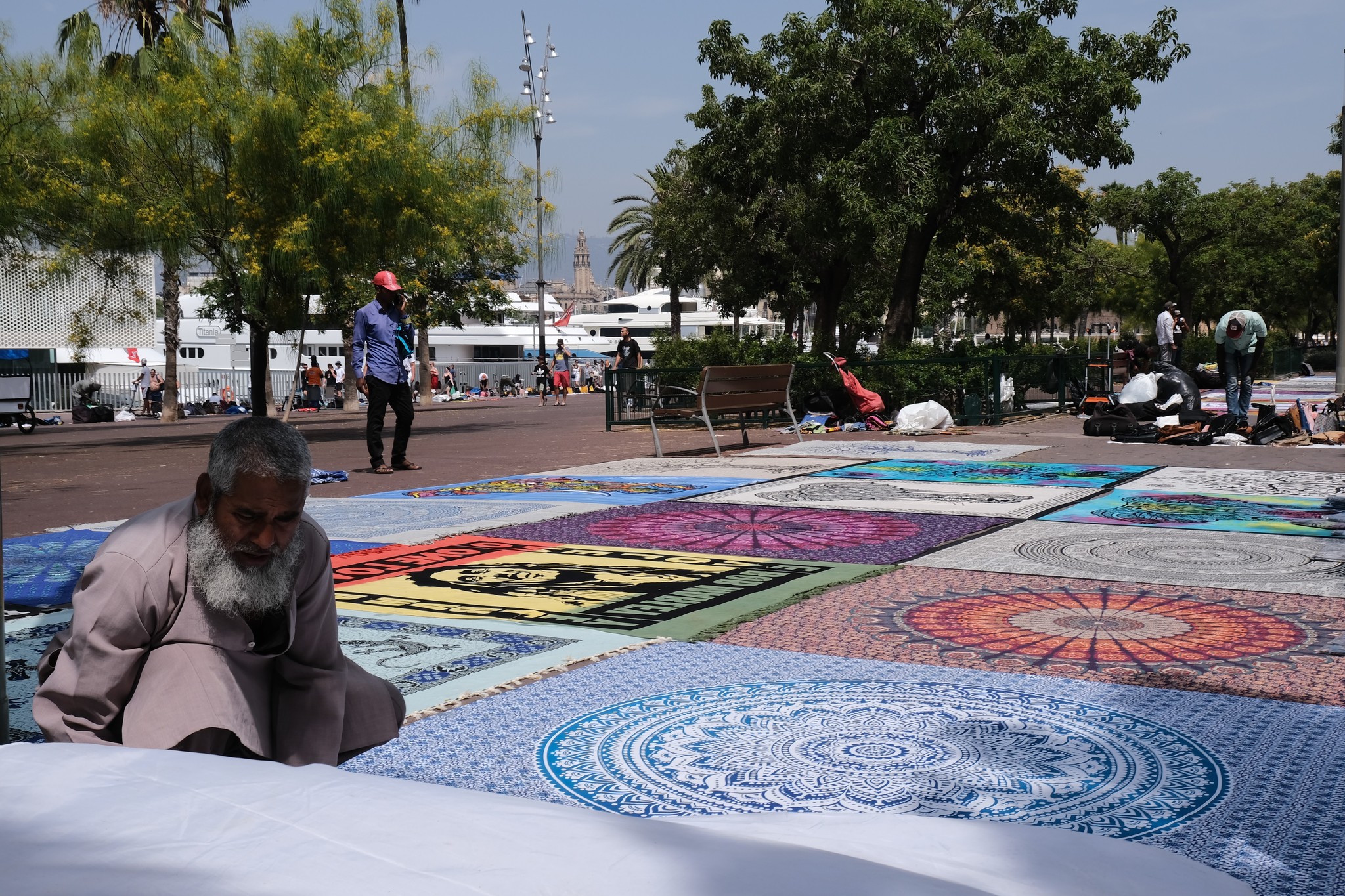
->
[1198,376,1345,415]
[1,438,1343,895]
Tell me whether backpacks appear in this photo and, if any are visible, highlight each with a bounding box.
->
[178,400,251,419]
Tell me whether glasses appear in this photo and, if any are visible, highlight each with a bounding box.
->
[576,362,579,363]
[586,363,588,364]
[445,369,447,370]
[429,364,433,365]
[150,371,155,373]
[304,367,307,368]
[329,367,333,368]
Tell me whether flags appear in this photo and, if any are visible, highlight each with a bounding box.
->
[549,305,574,326]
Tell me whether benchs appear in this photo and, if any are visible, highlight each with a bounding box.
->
[623,362,804,460]
[1080,350,1130,393]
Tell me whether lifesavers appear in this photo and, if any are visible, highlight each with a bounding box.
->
[223,389,233,402]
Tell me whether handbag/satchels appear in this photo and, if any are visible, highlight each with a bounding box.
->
[571,369,603,378]
[156,374,165,391]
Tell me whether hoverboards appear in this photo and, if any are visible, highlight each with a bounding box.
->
[122,381,160,419]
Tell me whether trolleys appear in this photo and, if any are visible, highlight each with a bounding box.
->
[1077,322,1111,415]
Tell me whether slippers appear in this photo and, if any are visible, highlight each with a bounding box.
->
[374,466,394,473]
[136,412,151,415]
[554,402,566,406]
[390,462,422,470]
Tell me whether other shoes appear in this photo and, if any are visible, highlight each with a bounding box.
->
[513,394,516,397]
[538,398,547,406]
[500,395,503,398]
[627,401,633,408]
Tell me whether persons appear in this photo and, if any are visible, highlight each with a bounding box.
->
[71,326,651,418]
[1114,301,1268,425]
[983,333,992,345]
[351,270,423,474]
[28,420,406,768]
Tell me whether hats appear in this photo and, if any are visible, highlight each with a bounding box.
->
[141,358,147,364]
[335,361,341,366]
[557,338,564,345]
[516,374,521,377]
[482,374,486,379]
[536,356,545,360]
[92,383,101,391]
[308,355,316,359]
[415,381,419,385]
[374,271,402,291]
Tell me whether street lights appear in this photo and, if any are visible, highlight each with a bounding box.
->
[521,13,558,401]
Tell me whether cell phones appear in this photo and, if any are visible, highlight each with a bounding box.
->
[393,294,400,304]
